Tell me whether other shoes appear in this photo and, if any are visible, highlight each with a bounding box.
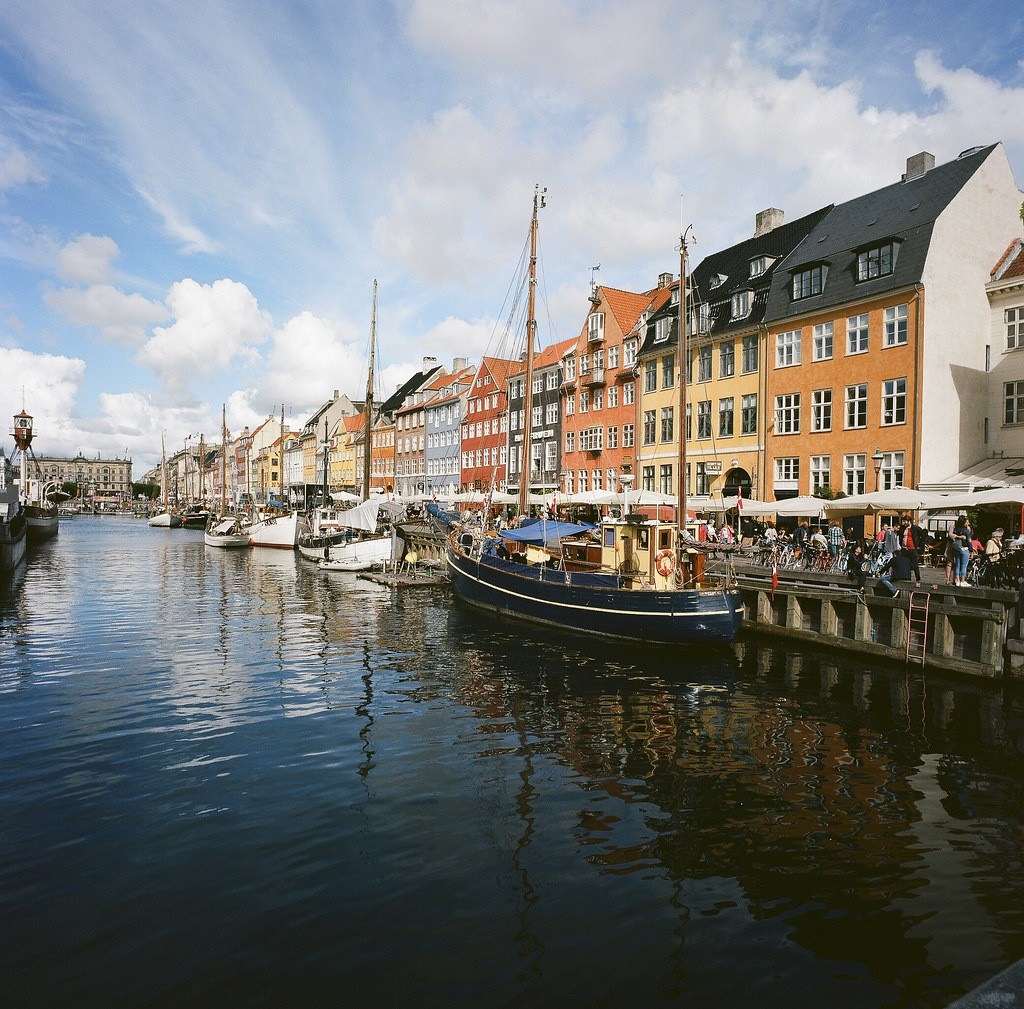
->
[858,587,865,593]
[946,581,950,584]
[951,581,954,584]
[714,556,718,560]
[915,582,921,588]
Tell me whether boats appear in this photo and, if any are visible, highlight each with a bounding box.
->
[19,503,60,527]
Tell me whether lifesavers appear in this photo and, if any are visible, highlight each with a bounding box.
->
[244,506,249,512]
[655,550,676,577]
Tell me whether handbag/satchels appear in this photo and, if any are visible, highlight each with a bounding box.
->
[951,537,962,551]
[848,570,855,581]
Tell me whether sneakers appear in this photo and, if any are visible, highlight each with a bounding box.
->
[892,590,900,598]
[961,581,972,587]
[956,582,961,586]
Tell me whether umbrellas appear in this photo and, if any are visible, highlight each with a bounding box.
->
[673,495,830,535]
[328,491,360,509]
[544,490,627,526]
[370,491,544,512]
[919,487,1024,539]
[818,489,950,543]
[596,489,678,526]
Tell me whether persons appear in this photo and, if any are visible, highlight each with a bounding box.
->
[948,515,974,588]
[707,515,1010,588]
[896,515,924,587]
[461,503,545,533]
[494,543,510,562]
[847,544,872,594]
[877,550,911,598]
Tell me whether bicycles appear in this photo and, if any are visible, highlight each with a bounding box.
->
[967,548,1019,591]
[751,533,879,575]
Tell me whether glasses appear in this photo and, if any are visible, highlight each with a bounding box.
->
[995,536,1002,538]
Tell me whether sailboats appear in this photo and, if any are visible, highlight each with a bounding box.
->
[422,181,747,652]
[144,278,407,571]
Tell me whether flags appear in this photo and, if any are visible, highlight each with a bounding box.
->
[738,486,743,510]
